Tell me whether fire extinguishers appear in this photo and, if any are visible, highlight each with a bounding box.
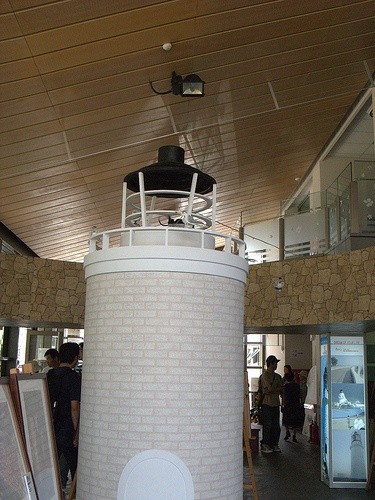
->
[309,419,319,443]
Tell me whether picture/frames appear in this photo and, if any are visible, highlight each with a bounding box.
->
[0,375,39,500]
[9,372,64,500]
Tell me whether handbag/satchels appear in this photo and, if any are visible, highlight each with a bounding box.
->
[259,404,272,415]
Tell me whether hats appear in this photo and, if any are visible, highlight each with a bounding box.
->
[298,371,308,379]
[266,356,280,363]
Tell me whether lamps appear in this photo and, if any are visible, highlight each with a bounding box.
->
[150,71,206,98]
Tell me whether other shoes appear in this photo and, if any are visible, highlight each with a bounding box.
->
[271,444,281,451]
[284,430,291,440]
[292,435,297,441]
[261,443,273,452]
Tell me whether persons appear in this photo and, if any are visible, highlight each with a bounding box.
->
[258,364,308,443]
[259,355,284,453]
[42,342,84,499]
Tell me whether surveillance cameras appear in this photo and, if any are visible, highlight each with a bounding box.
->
[276,284,283,290]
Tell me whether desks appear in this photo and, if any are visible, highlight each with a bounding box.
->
[242,425,261,455]
